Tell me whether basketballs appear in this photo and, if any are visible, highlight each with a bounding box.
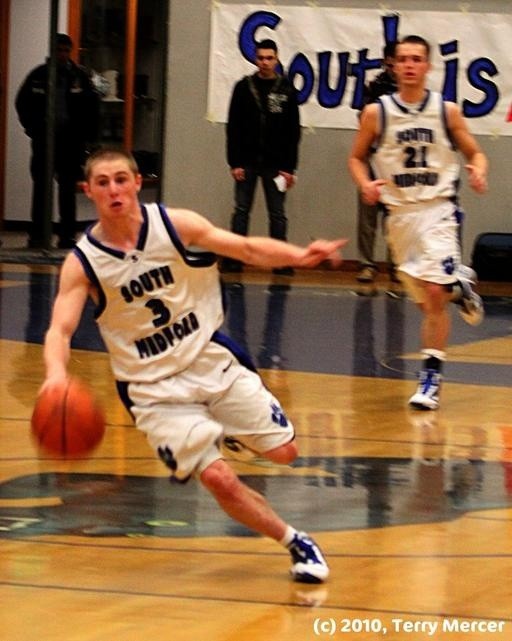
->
[31,377,104,458]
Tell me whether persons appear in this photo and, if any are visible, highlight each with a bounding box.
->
[14,33,107,252]
[346,33,490,412]
[39,148,348,585]
[353,40,402,282]
[214,41,300,278]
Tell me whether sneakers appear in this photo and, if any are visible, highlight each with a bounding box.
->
[453,280,485,327]
[288,529,330,585]
[408,370,442,409]
[355,266,378,281]
